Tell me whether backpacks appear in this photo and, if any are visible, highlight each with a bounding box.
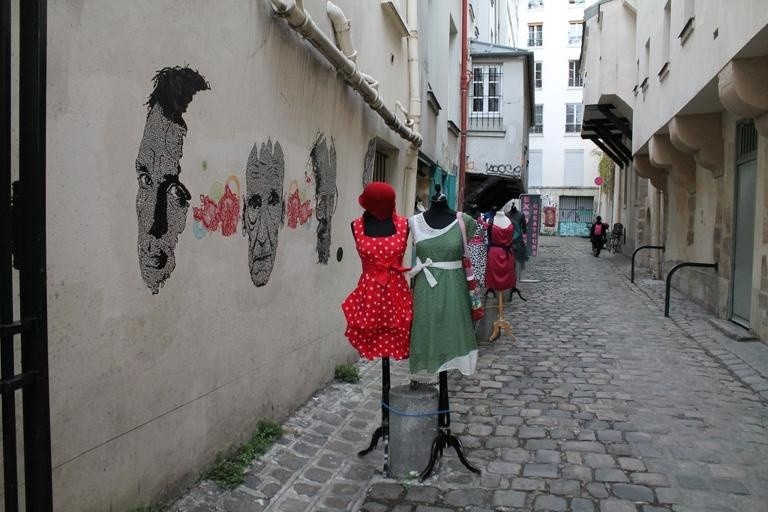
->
[594,223,602,235]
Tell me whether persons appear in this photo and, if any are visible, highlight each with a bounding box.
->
[341,181,414,361]
[304,132,338,264]
[486,209,517,292]
[134,65,211,294]
[241,138,285,287]
[590,215,606,257]
[406,195,477,376]
[504,206,530,263]
[463,222,487,288]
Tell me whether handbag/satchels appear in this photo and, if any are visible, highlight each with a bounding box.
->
[463,256,484,319]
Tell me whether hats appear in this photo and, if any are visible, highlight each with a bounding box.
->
[358,181,396,220]
